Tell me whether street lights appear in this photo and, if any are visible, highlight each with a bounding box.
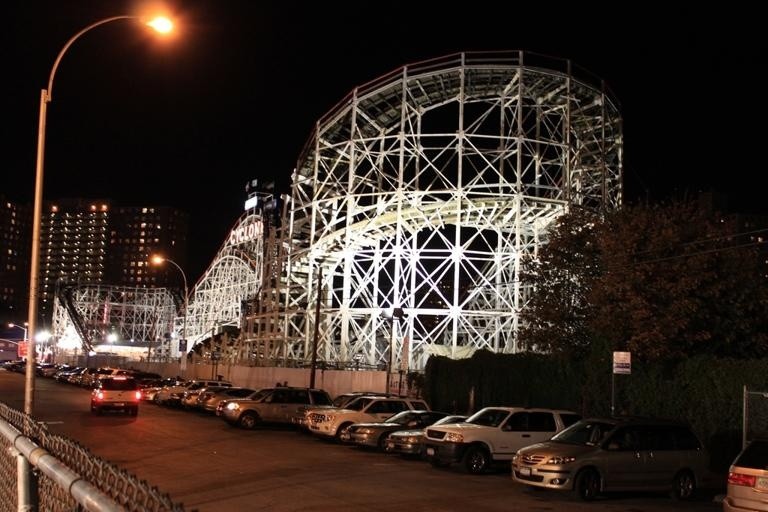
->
[24,14,177,439]
[9,322,27,362]
[153,257,189,378]
[742,383,767,447]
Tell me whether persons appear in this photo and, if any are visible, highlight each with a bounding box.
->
[274,382,281,387]
[283,380,289,387]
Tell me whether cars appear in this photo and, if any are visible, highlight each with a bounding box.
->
[722,436,768,512]
